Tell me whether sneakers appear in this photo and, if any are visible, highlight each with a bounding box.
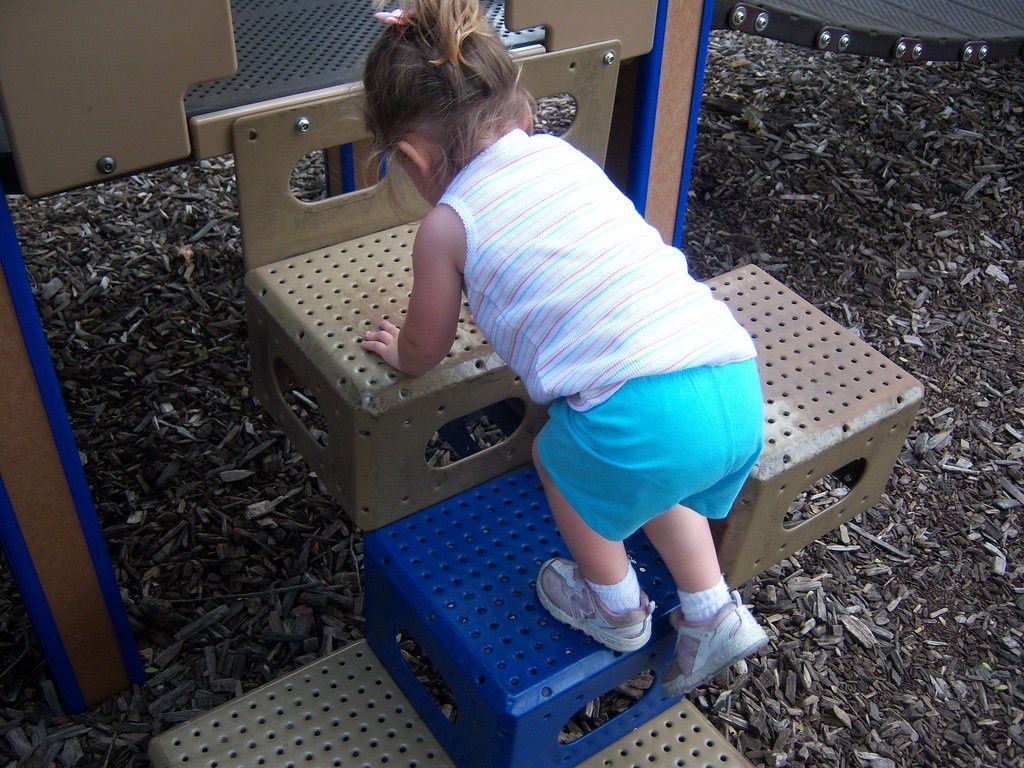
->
[661,589,769,697]
[536,557,656,653]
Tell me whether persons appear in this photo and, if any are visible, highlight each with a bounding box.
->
[357,0,769,696]
